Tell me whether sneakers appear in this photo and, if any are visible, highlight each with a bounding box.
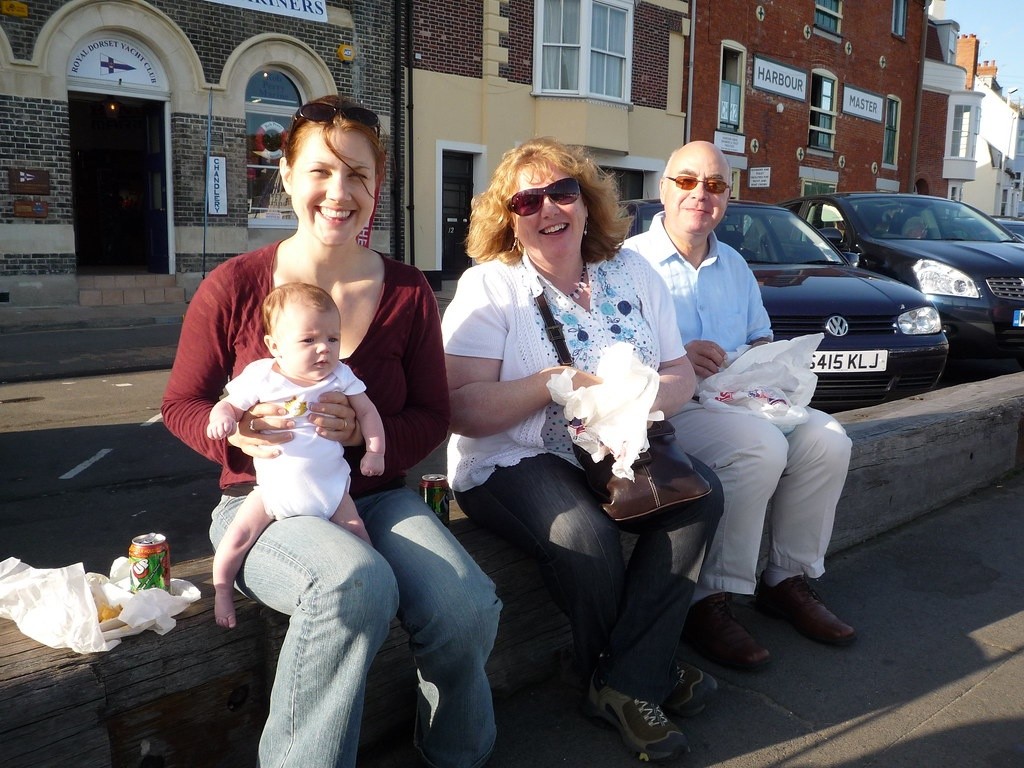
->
[586,670,690,764]
[665,663,717,717]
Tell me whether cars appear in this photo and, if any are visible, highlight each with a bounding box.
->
[618,198,949,413]
[742,191,1024,370]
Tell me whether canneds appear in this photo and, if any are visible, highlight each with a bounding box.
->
[129,533,171,595]
[419,474,449,527]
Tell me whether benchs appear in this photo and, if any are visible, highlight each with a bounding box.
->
[0,372,1023,768]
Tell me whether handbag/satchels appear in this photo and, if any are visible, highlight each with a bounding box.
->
[572,417,714,535]
[702,332,825,434]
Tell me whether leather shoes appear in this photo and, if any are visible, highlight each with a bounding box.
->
[757,568,856,646]
[681,601,771,675]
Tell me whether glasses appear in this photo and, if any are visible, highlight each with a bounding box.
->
[508,178,581,217]
[664,174,733,195]
[287,102,382,157]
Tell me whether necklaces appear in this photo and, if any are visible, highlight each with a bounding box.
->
[566,258,586,298]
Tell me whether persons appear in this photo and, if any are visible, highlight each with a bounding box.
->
[900,215,928,240]
[161,96,514,768]
[618,137,855,668]
[440,138,724,768]
[207,283,386,626]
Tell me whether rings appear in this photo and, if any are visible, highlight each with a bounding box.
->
[248,419,255,431]
[344,420,347,428]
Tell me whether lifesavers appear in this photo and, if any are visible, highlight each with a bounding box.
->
[255,120,288,160]
